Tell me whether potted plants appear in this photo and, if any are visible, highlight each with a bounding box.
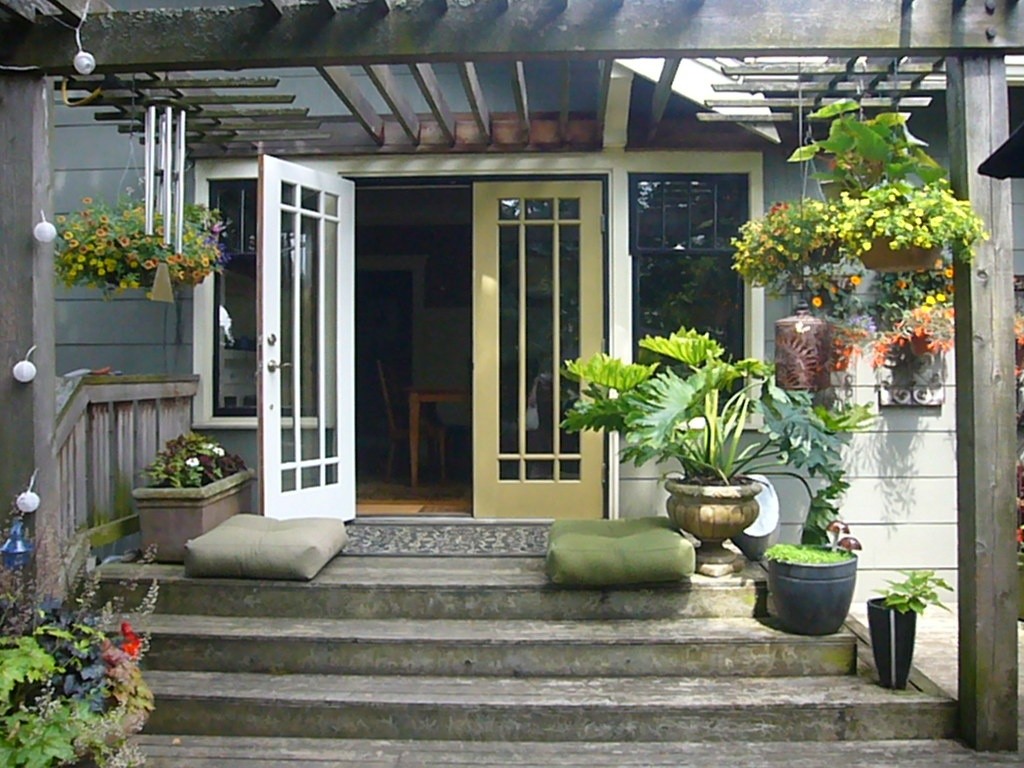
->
[559,326,883,544]
[762,521,862,637]
[867,572,954,692]
[131,431,252,561]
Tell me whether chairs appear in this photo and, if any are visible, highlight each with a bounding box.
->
[376,359,445,484]
[501,359,540,435]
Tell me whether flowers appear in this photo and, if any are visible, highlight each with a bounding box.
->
[731,97,991,299]
[0,473,159,768]
[797,259,956,371]
[49,191,231,304]
[1013,312,1024,554]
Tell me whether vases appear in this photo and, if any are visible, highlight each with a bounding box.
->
[860,237,942,272]
[775,310,830,389]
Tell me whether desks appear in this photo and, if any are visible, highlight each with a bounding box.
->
[402,388,470,489]
